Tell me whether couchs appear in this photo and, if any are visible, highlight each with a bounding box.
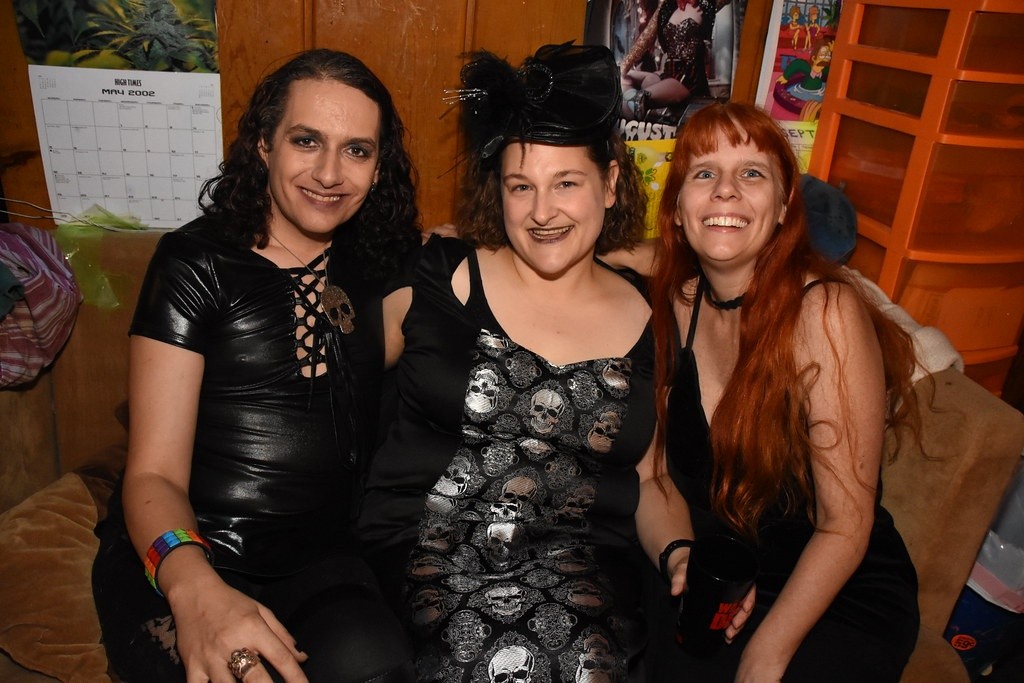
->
[0,217,1024,683]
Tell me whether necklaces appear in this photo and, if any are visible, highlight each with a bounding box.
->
[702,278,750,310]
[268,233,356,334]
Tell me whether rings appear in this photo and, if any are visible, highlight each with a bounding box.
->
[228,648,261,679]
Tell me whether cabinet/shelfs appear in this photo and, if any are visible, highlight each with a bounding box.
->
[808,0,1024,401]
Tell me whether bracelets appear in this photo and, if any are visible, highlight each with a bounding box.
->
[660,539,694,587]
[144,528,213,595]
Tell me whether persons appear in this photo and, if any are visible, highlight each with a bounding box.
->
[358,38,759,683]
[93,48,461,683]
[619,1,736,112]
[591,102,952,682]
